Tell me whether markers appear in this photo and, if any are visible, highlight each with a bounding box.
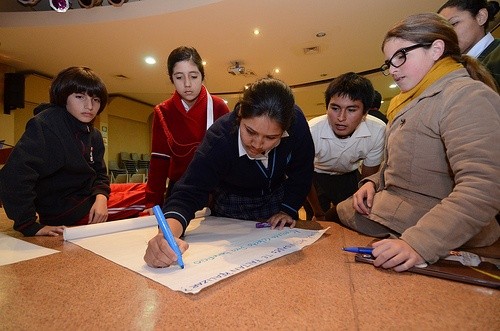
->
[153,205,187,270]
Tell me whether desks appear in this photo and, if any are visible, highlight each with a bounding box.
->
[0,221,500,331]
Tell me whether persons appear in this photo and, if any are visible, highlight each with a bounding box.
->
[436,0,500,94]
[326,12,500,273]
[143,72,315,270]
[0,65,146,238]
[142,44,230,216]
[303,70,387,221]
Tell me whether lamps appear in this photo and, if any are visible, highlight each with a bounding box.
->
[18,0,128,13]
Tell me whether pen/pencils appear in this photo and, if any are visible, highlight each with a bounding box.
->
[256,221,293,229]
[340,246,374,255]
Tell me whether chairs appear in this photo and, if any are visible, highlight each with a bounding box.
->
[108,152,150,184]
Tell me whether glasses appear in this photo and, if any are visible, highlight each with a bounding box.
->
[380,43,432,76]
